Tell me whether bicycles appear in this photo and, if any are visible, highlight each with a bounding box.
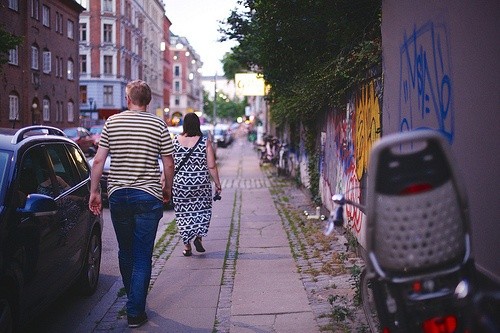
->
[257,132,294,181]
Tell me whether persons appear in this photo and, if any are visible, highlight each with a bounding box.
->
[161,113,222,255]
[89,80,175,328]
[37,175,86,231]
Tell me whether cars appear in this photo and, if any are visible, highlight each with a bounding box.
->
[89,125,106,149]
[168,123,239,150]
[61,127,95,156]
[87,153,111,205]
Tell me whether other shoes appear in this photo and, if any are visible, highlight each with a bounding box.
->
[183,250,192,255]
[128,312,149,327]
[194,237,206,252]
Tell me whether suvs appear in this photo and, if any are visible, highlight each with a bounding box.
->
[0,124,103,333]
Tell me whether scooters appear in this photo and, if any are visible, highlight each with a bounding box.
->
[330,130,485,333]
